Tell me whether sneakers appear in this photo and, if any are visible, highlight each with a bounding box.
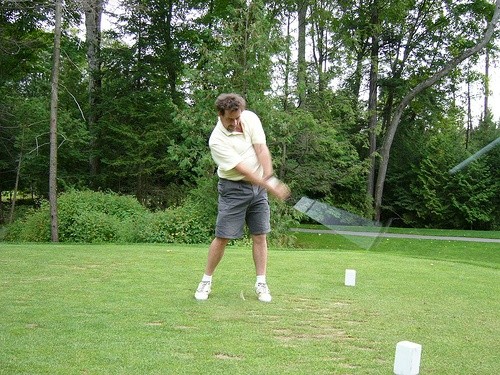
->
[254,279,273,303]
[194,281,214,300]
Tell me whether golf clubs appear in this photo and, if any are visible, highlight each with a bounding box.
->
[284,196,393,250]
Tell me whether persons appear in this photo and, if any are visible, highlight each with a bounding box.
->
[193,93,290,302]
